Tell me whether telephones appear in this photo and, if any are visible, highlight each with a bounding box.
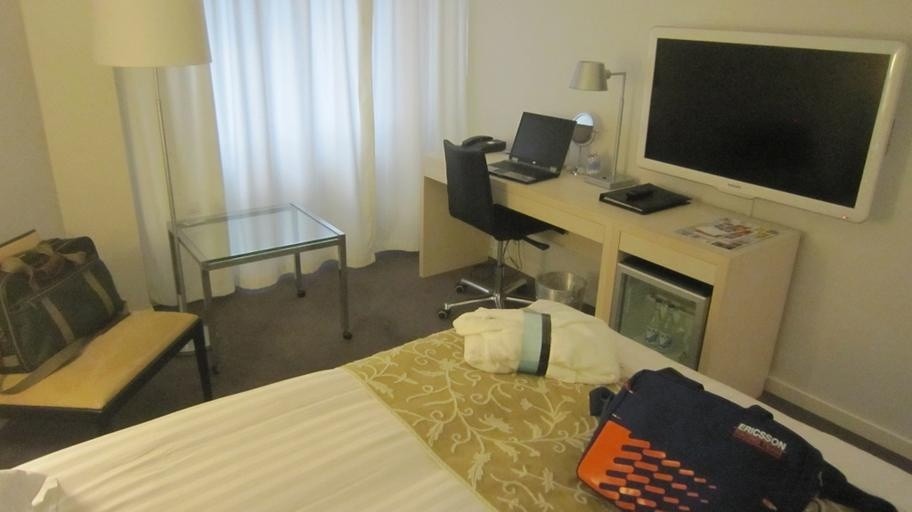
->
[461,135,506,153]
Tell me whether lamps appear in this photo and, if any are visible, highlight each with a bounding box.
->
[568,59,641,189]
[93,0,214,354]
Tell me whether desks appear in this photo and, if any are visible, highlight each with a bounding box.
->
[419,147,805,400]
[163,202,353,373]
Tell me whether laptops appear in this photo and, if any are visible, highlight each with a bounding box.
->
[487,112,577,184]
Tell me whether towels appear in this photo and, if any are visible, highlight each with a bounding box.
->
[453,306,625,385]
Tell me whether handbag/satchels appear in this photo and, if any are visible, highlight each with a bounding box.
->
[0,236,128,373]
[576,367,845,512]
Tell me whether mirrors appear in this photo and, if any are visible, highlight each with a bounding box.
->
[568,112,600,175]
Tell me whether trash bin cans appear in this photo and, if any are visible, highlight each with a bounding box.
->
[535,271,588,311]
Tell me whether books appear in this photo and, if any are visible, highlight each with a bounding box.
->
[596,183,692,217]
[673,216,780,251]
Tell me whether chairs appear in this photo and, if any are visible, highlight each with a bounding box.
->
[0,228,214,441]
[437,139,548,318]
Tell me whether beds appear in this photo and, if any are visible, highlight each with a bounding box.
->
[1,300,911,512]
